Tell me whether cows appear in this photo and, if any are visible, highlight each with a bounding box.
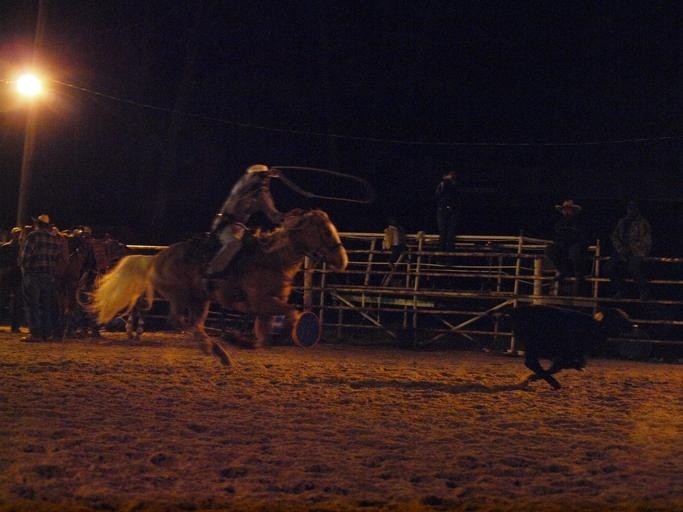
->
[492,302,639,393]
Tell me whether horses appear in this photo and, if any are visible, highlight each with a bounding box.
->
[74,204,349,371]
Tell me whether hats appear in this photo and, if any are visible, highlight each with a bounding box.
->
[30,214,50,229]
[555,200,582,213]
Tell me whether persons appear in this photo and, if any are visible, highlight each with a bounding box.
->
[550,197,583,280]
[204,164,303,282]
[0,214,115,344]
[432,165,466,255]
[602,202,654,302]
